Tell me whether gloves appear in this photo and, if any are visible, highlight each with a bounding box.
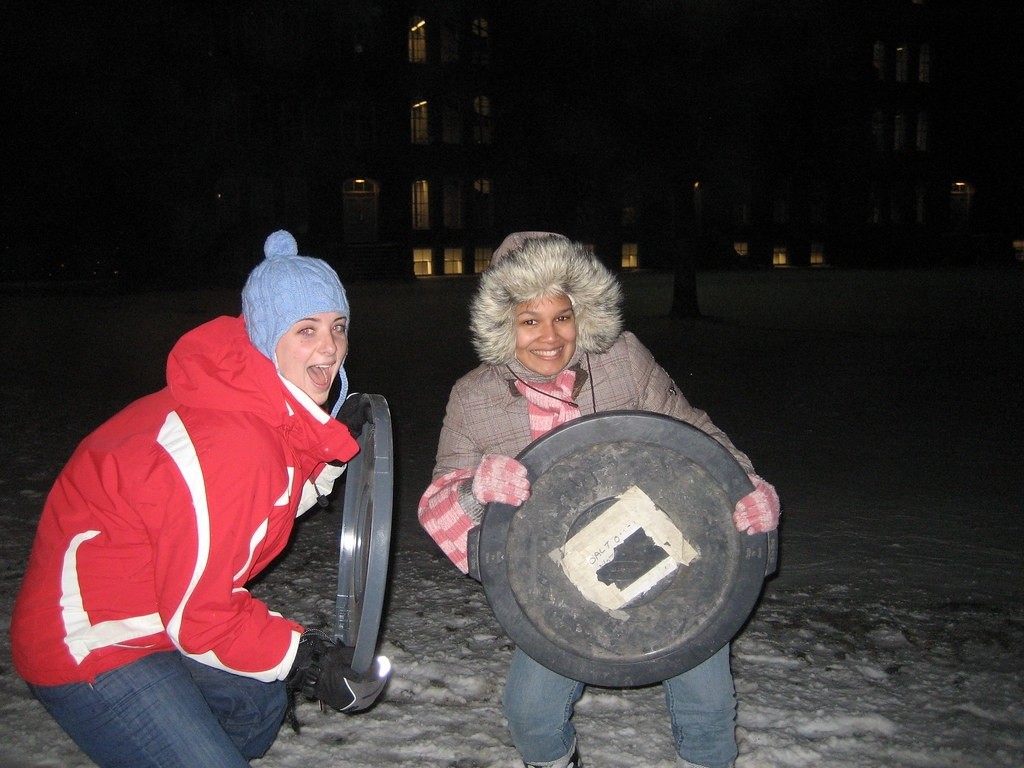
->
[282,631,387,733]
[327,388,388,467]
[732,472,780,535]
[472,455,531,508]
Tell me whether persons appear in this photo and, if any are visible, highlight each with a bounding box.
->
[10,230,370,767]
[418,232,781,768]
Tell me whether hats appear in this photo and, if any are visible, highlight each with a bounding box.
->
[242,229,350,364]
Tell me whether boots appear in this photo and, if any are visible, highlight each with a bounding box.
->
[523,737,582,768]
